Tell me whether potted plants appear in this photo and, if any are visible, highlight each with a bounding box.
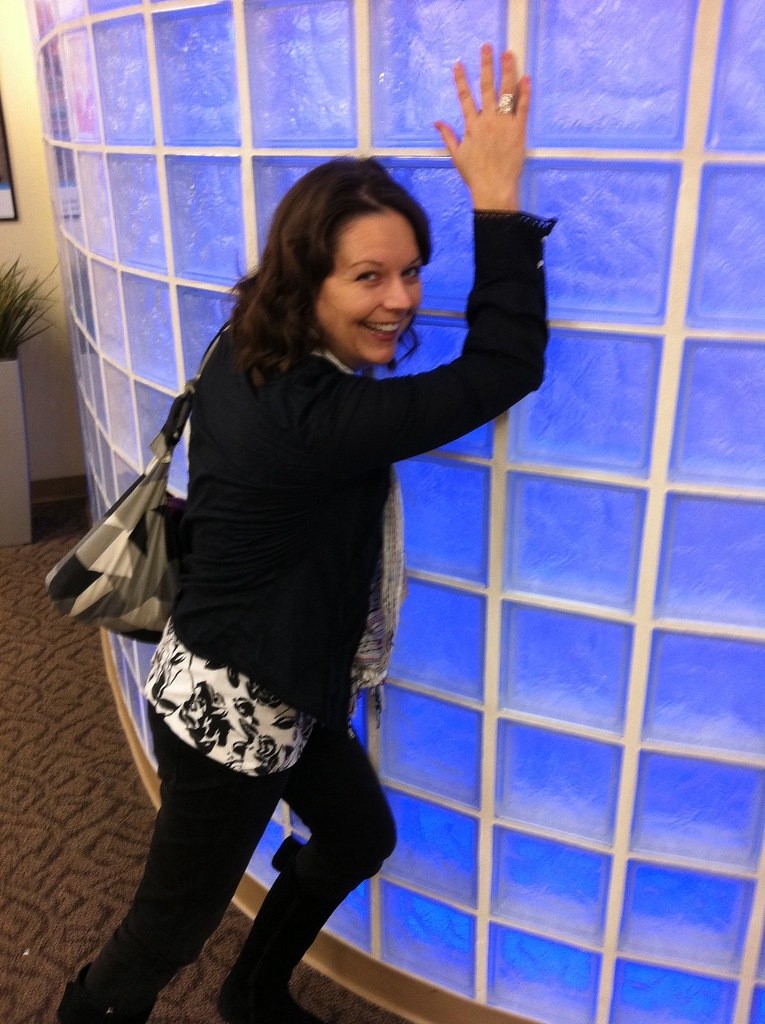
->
[0,252,59,548]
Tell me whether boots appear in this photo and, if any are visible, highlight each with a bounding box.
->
[218,940,325,1024]
[55,967,154,1024]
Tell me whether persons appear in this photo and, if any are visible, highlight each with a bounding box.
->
[55,41,557,1024]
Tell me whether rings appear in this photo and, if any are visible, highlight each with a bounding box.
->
[497,92,515,115]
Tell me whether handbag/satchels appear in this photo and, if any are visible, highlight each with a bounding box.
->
[43,318,237,645]
